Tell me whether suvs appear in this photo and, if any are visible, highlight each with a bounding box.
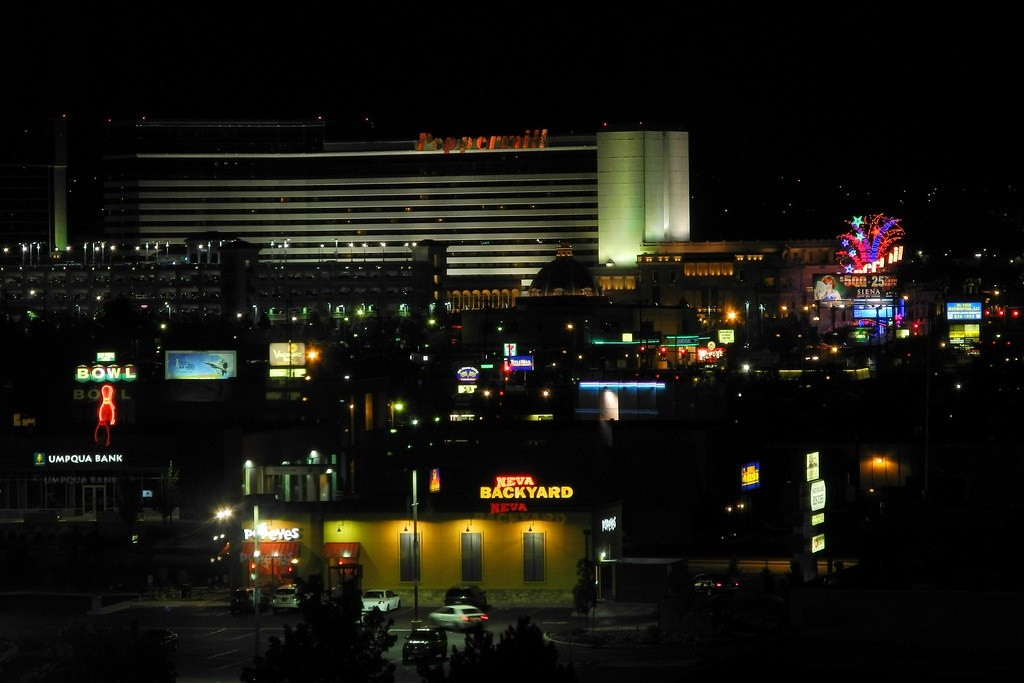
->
[232,585,264,614]
[443,582,488,611]
[273,581,302,614]
[402,624,449,664]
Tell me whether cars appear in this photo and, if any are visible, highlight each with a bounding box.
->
[426,603,489,632]
[362,588,402,613]
